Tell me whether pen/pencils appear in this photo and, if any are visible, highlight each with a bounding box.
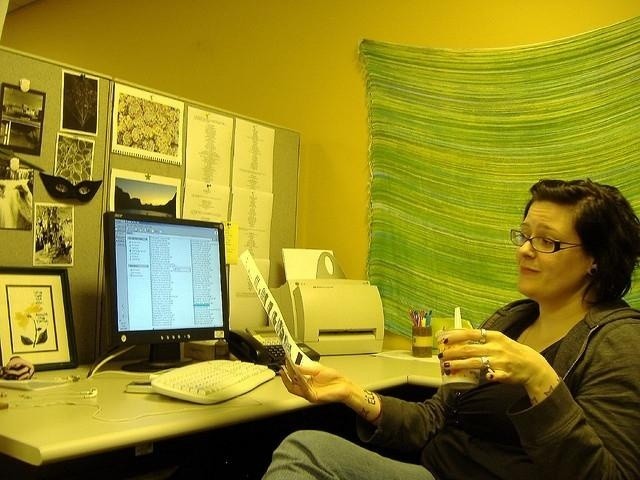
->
[409,308,432,327]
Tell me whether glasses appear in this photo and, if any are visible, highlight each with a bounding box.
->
[510,229,583,254]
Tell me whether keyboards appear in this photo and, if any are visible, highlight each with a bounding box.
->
[149,358,277,405]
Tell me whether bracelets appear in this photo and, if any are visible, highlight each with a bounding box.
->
[371,408,384,422]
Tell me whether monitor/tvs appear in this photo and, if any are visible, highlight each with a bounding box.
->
[103,211,230,372]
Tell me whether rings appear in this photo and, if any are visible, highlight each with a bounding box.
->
[481,354,490,372]
[479,328,490,343]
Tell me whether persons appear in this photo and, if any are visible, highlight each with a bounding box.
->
[259,177,640,480]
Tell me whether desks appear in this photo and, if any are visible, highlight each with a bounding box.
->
[0,350,449,471]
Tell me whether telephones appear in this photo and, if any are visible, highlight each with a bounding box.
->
[225,325,321,376]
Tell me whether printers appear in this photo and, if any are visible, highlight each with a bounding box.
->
[265,251,385,356]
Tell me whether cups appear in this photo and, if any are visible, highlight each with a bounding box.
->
[438,329,481,390]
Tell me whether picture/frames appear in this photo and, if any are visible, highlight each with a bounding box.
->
[0,266,79,373]
